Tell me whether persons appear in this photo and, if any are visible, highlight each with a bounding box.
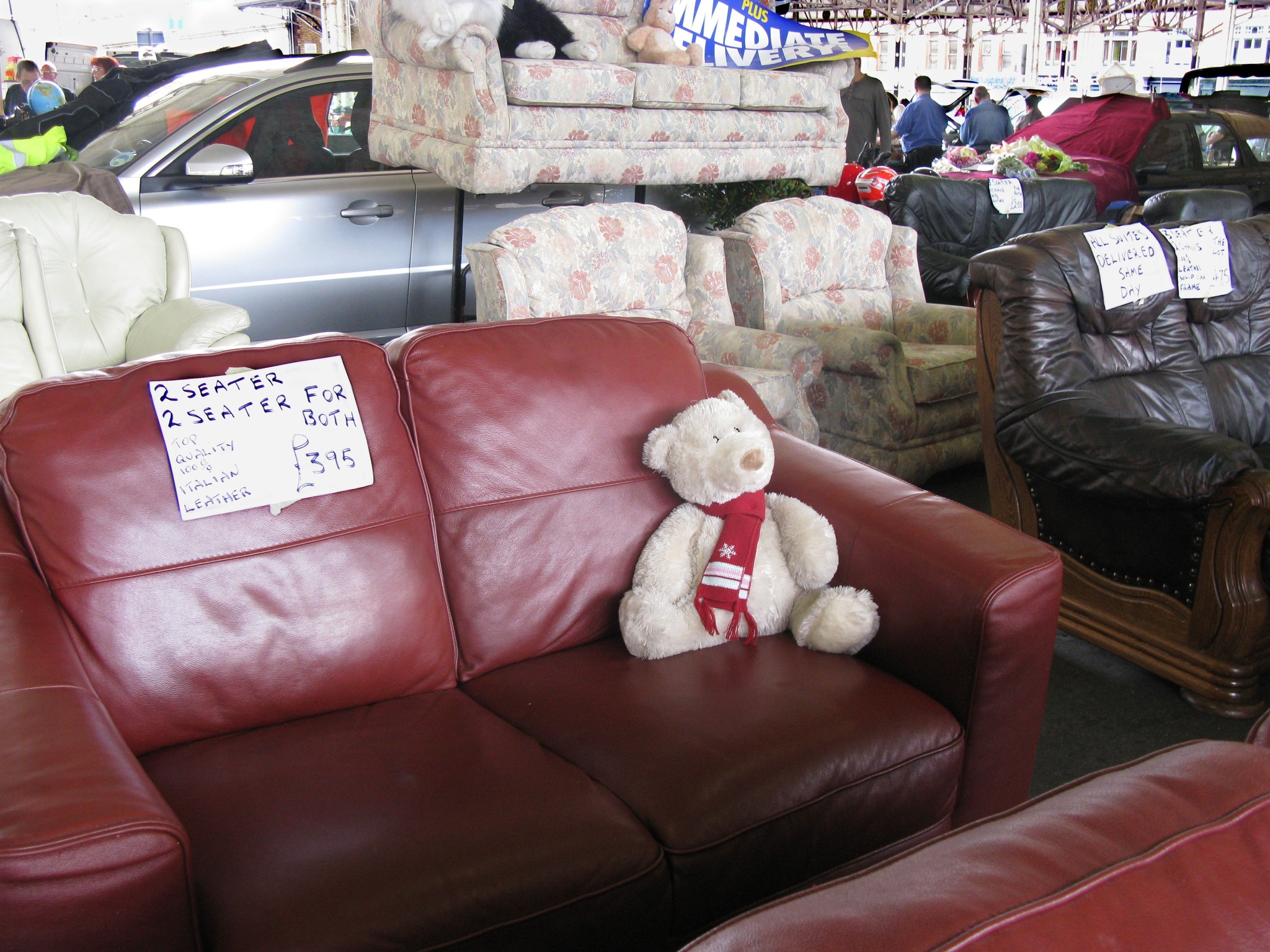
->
[3,59,41,117]
[90,57,119,82]
[875,96,900,145]
[1013,93,1044,135]
[899,98,911,108]
[39,64,77,104]
[840,56,893,169]
[1206,125,1233,161]
[960,86,1014,156]
[1248,138,1268,161]
[886,91,907,121]
[892,76,948,166]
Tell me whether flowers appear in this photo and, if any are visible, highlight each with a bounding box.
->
[991,151,1037,180]
[931,143,982,174]
[1012,134,1090,176]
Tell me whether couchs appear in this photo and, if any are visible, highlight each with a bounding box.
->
[677,735,1270,952]
[0,161,253,410]
[355,0,857,196]
[0,311,1069,952]
[464,173,1270,721]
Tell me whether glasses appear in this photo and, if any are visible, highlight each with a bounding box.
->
[92,68,105,73]
[18,76,38,87]
[40,70,57,75]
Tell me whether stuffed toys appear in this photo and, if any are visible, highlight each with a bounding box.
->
[626,0,703,67]
[391,0,598,61]
[618,389,879,656]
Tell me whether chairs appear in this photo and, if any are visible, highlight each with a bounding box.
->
[254,82,421,181]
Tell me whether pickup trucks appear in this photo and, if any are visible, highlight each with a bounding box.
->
[39,47,687,350]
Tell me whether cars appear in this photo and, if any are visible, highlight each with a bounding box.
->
[1128,106,1270,212]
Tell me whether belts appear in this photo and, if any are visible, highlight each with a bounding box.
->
[913,146,942,152]
[979,150,986,153]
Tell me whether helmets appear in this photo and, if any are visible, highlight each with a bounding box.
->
[910,167,941,178]
[828,163,866,203]
[855,166,898,207]
